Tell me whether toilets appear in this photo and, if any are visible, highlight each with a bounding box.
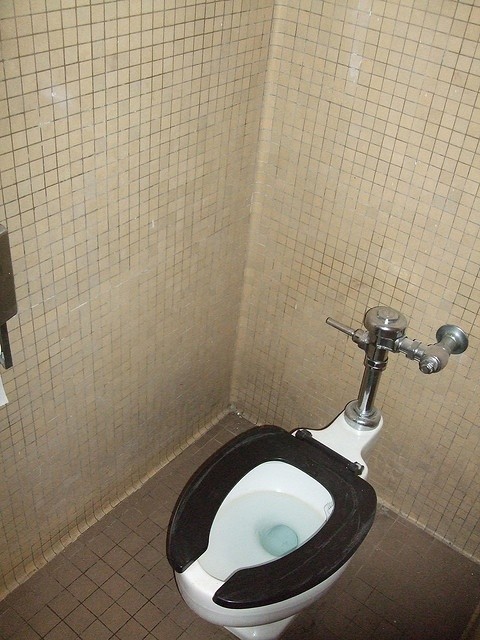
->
[166,400,383,640]
